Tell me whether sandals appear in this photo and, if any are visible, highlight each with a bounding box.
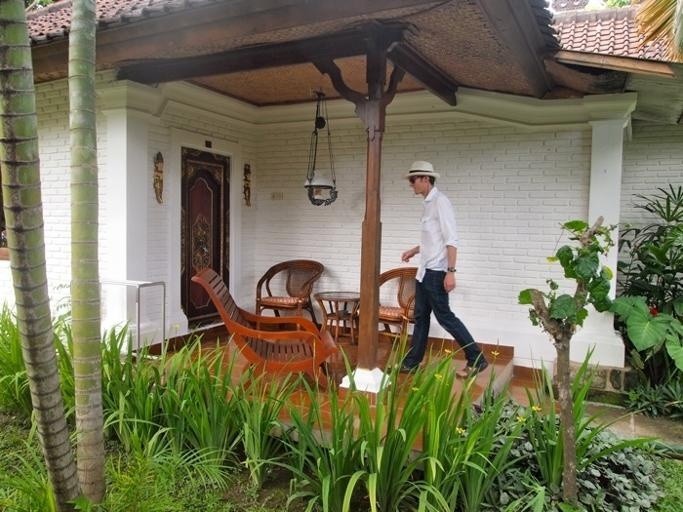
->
[385,362,416,374]
[456,361,488,377]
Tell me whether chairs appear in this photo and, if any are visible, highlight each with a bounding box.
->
[191,258,420,397]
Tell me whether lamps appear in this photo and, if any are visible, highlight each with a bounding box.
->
[302,88,341,206]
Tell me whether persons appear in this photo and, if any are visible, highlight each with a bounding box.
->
[385,161,488,378]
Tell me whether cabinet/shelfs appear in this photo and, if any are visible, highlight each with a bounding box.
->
[101,278,166,369]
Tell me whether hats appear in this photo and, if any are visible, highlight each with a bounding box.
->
[404,160,440,181]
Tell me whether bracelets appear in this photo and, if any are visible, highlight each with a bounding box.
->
[447,267,456,272]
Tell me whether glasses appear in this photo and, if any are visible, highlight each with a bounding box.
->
[411,176,416,182]
